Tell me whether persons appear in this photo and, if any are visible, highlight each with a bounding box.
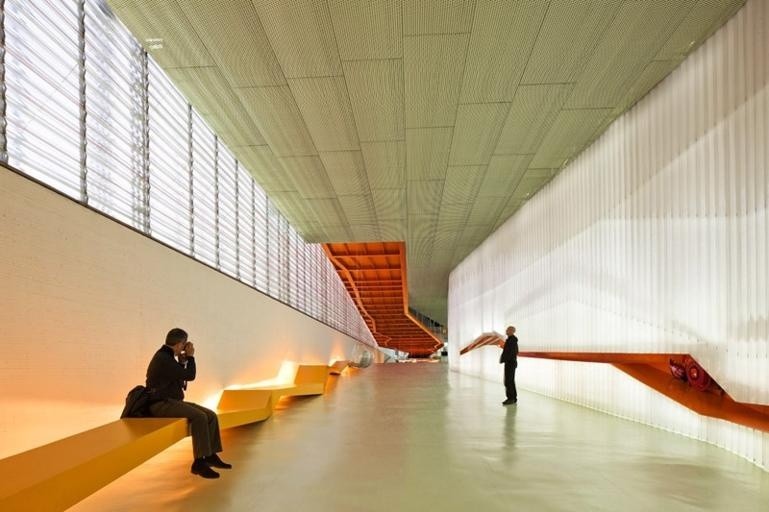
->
[145,327,232,479]
[499,326,519,405]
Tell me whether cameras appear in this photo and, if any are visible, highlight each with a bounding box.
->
[182,342,189,350]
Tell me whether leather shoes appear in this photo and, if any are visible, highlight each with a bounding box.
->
[191,461,219,478]
[204,455,231,468]
[503,400,514,404]
